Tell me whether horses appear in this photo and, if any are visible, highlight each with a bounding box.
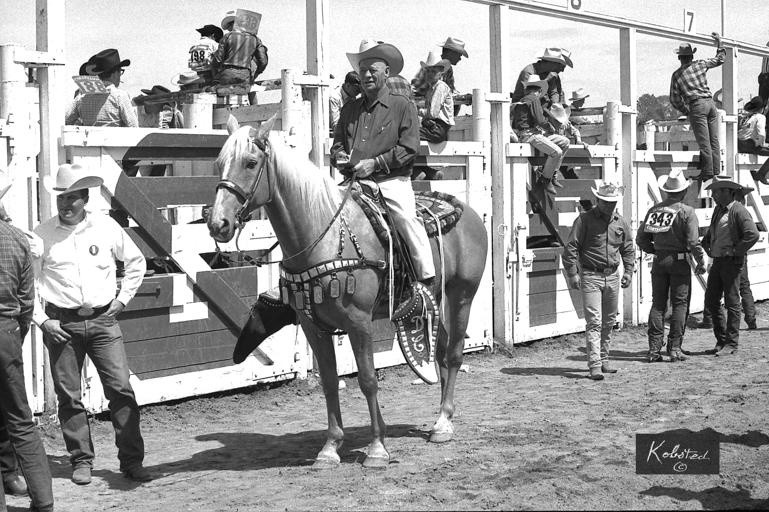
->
[206,110,489,471]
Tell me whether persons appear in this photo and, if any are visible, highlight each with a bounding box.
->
[31,164,154,484]
[636,167,706,362]
[64,8,768,298]
[694,193,759,329]
[0,169,52,511]
[562,182,635,379]
[702,176,760,356]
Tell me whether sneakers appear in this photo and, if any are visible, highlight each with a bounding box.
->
[28,502,54,512]
[3,476,29,499]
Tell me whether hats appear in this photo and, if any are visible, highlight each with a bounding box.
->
[41,162,104,196]
[544,102,571,124]
[196,24,223,41]
[590,178,628,202]
[520,74,548,97]
[345,36,405,78]
[657,169,693,193]
[672,42,698,55]
[436,36,469,58]
[703,174,743,192]
[743,96,768,112]
[221,10,237,31]
[78,47,132,79]
[561,46,574,68]
[536,47,566,66]
[141,85,174,107]
[420,50,451,74]
[568,87,591,101]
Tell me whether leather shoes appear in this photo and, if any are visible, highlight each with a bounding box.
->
[601,362,617,374]
[690,172,714,182]
[666,349,691,362]
[552,171,564,188]
[72,464,93,485]
[705,342,724,354]
[589,366,605,380]
[120,465,153,483]
[715,344,738,357]
[537,173,557,196]
[647,349,677,362]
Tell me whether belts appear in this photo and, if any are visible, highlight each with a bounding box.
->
[582,264,619,275]
[46,303,112,318]
